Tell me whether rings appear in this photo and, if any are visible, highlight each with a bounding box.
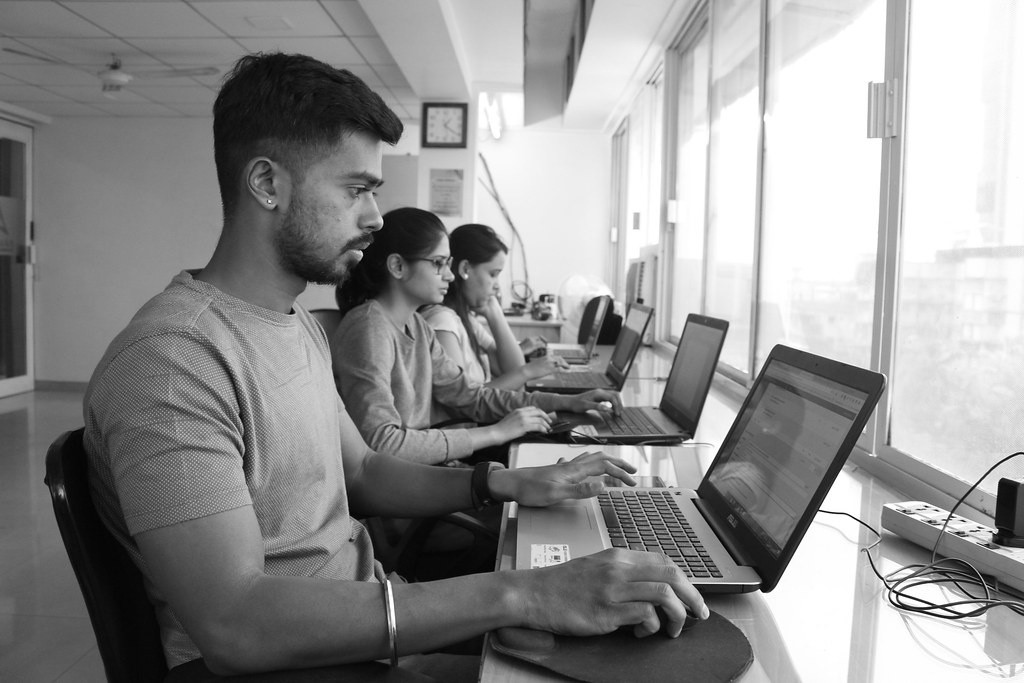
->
[554,361,558,366]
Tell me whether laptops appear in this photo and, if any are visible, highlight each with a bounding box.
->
[513,294,888,592]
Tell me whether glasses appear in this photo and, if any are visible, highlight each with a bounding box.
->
[406,256,454,268]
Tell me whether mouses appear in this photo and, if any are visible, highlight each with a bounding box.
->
[648,597,700,633]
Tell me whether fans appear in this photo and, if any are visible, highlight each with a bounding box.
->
[2,37,222,92]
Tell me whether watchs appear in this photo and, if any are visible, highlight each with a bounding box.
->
[473,461,505,507]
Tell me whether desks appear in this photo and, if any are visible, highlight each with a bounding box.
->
[563,313,1024,683]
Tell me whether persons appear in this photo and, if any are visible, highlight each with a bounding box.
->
[331,206,623,463]
[83,54,710,677]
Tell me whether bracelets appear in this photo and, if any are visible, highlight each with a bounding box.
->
[383,580,399,669]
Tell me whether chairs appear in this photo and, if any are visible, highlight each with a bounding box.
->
[43,425,210,683]
[306,309,499,581]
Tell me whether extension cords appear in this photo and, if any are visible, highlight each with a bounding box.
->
[881,500,1024,593]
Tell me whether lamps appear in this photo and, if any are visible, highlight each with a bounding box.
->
[421,102,468,149]
